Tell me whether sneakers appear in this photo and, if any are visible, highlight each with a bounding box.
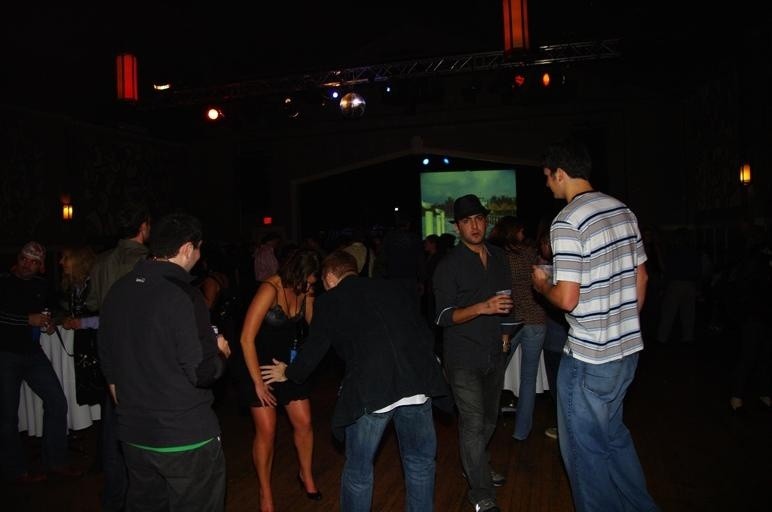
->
[491,471,505,486]
[469,494,501,512]
[545,427,558,439]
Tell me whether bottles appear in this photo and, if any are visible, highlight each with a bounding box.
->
[40,308,51,333]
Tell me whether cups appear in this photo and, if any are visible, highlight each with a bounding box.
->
[536,265,553,286]
[496,289,511,312]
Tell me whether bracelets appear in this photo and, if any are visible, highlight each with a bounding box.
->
[502,341,510,345]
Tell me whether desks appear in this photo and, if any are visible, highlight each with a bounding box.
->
[18,325,103,438]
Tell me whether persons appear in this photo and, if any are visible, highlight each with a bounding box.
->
[1,196,570,512]
[639,218,770,419]
[534,141,659,510]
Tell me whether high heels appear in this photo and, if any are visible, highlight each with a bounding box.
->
[297,474,322,500]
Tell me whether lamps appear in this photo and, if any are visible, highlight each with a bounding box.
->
[502,0,531,56]
[115,49,140,113]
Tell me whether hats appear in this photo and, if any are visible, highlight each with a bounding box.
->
[450,195,490,224]
[21,241,45,261]
